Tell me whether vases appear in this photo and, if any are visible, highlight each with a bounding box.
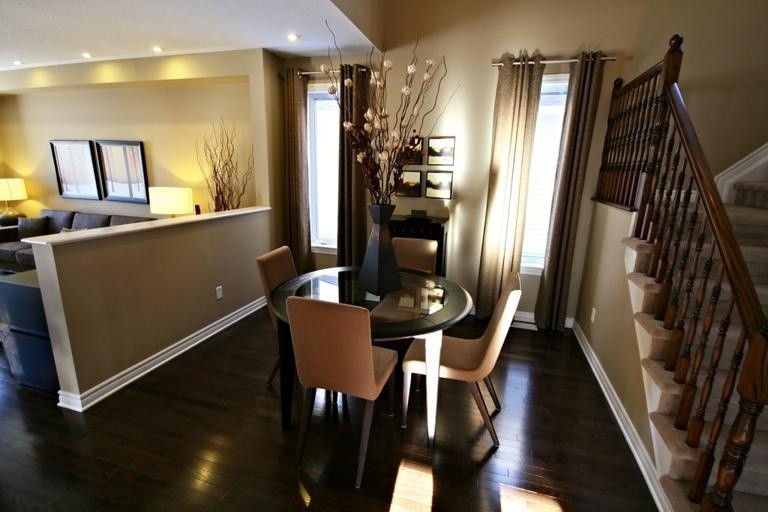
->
[356,205,402,296]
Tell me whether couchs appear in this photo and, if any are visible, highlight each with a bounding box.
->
[0,209,177,394]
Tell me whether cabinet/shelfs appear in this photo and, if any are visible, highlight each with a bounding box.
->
[389,215,450,277]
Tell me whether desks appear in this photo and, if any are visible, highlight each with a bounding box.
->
[268,266,473,448]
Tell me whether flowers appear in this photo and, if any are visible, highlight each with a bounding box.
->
[323,19,462,204]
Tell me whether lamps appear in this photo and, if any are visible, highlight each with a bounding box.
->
[148,187,194,218]
[0,178,28,226]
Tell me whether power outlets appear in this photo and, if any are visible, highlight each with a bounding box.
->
[215,286,223,299]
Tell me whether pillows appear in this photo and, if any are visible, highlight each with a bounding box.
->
[17,216,51,241]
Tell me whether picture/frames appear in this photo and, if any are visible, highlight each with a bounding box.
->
[427,136,455,166]
[402,137,423,164]
[395,170,422,197]
[425,170,452,199]
[95,140,150,204]
[50,141,101,200]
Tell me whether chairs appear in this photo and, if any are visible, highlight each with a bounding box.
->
[256,246,331,401]
[285,297,397,489]
[402,271,522,452]
